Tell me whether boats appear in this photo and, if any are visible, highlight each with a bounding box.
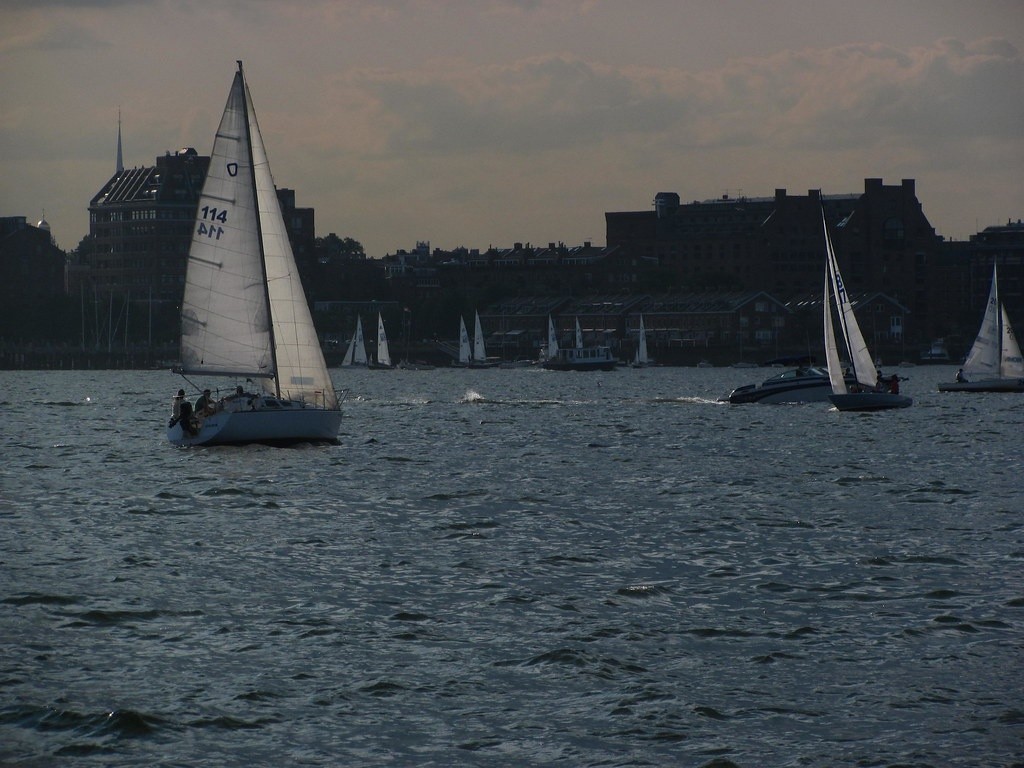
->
[728,364,908,406]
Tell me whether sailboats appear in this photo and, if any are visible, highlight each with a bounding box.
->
[340,312,663,374]
[819,188,913,412]
[938,252,1024,394]
[165,59,349,448]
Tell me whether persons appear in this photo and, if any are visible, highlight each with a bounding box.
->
[956,369,964,382]
[195,389,212,411]
[796,365,802,377]
[891,374,900,395]
[172,389,186,418]
[844,368,851,375]
